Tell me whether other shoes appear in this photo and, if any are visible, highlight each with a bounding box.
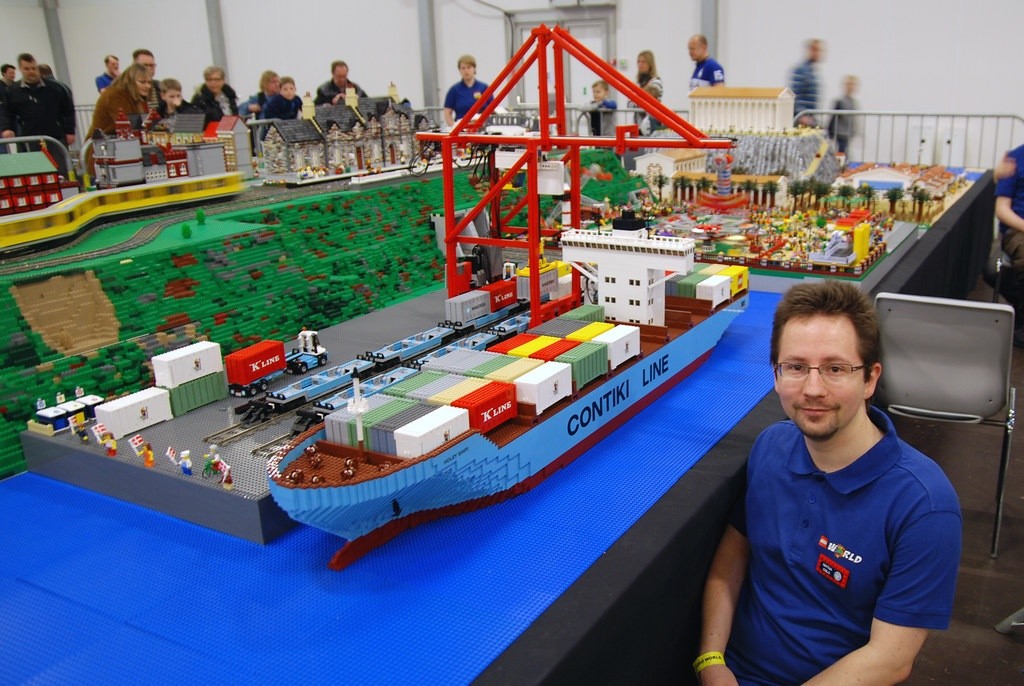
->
[1013,325,1024,347]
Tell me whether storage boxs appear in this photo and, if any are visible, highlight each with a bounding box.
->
[94,340,287,440]
[665,263,748,308]
[324,267,640,459]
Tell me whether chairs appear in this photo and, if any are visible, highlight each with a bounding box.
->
[991,167,1024,350]
[874,292,1015,557]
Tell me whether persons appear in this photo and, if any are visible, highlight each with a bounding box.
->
[132,49,160,109]
[698,279,964,686]
[790,39,827,128]
[83,63,151,142]
[829,74,860,158]
[636,50,663,137]
[0,53,76,177]
[71,423,234,490]
[96,55,119,94]
[588,79,617,136]
[314,61,368,106]
[159,78,191,118]
[444,54,494,128]
[994,144,1024,319]
[239,70,281,140]
[688,35,724,92]
[191,66,238,129]
[265,76,303,127]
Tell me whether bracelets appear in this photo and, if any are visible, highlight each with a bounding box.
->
[693,650,725,674]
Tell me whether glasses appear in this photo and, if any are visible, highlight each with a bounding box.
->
[773,361,869,385]
[141,63,156,69]
[205,78,221,83]
